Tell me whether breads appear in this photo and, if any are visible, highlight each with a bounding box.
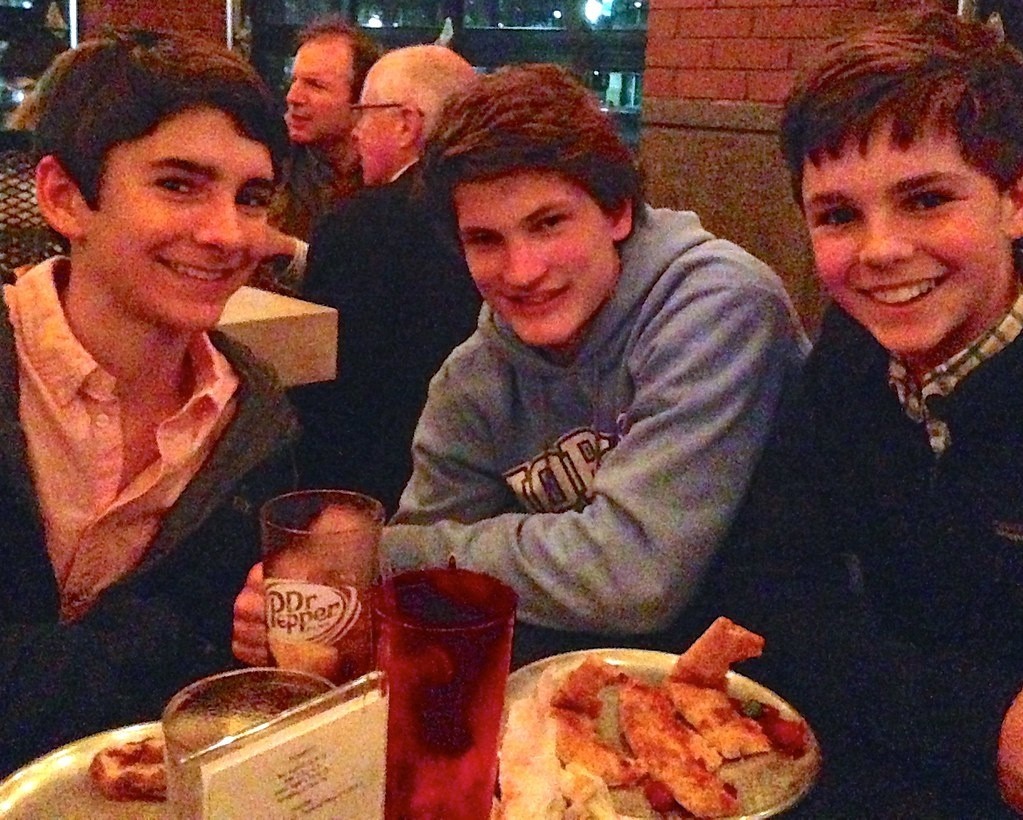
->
[87,736,165,803]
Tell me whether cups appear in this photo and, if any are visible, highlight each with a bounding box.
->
[259,489,385,690]
[369,566,516,820]
[163,667,337,820]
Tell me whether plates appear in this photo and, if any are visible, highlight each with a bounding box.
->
[0,719,165,819]
[506,646,822,820]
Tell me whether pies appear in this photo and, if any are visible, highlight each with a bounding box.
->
[548,613,770,820]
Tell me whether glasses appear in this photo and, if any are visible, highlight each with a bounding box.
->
[347,102,426,124]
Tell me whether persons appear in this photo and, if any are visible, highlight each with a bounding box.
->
[0,29,69,98]
[0,28,313,781]
[243,21,379,302]
[290,46,482,525]
[380,11,1023,820]
[231,64,814,668]
